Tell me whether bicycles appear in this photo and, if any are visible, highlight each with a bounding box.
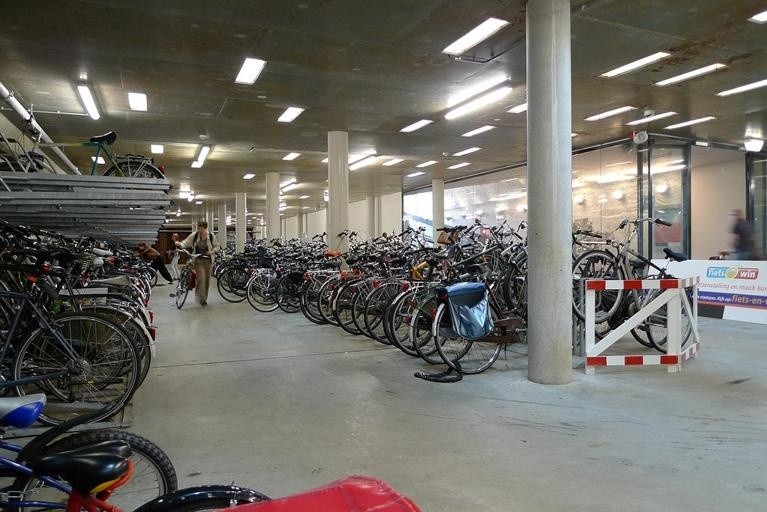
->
[169,247,211,308]
[0,393,178,511]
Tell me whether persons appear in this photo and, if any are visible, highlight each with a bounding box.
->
[135,243,173,284]
[167,233,182,280]
[728,209,753,261]
[175,221,221,305]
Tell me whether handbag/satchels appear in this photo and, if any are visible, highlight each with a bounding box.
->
[448,287,495,341]
